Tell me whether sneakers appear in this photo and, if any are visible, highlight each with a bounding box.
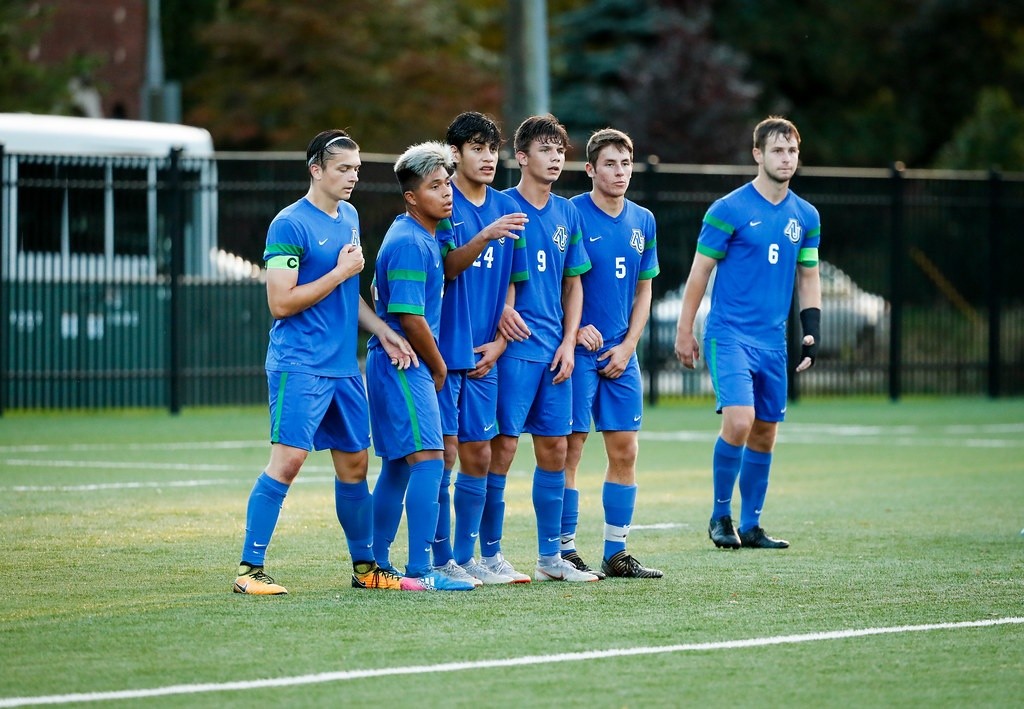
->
[737,526,790,549]
[233,568,288,594]
[561,551,606,580]
[460,557,514,584]
[479,552,531,583]
[351,560,405,590]
[601,550,663,578]
[431,558,483,586]
[708,515,742,550]
[400,570,476,591]
[535,553,599,583]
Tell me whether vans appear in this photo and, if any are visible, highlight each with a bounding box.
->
[0,112,223,286]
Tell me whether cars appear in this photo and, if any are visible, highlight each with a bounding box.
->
[640,260,895,376]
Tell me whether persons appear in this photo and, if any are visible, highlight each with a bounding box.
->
[561,127,662,580]
[501,111,598,583]
[673,117,822,552]
[435,107,531,589]
[225,125,420,596]
[364,141,474,591]
